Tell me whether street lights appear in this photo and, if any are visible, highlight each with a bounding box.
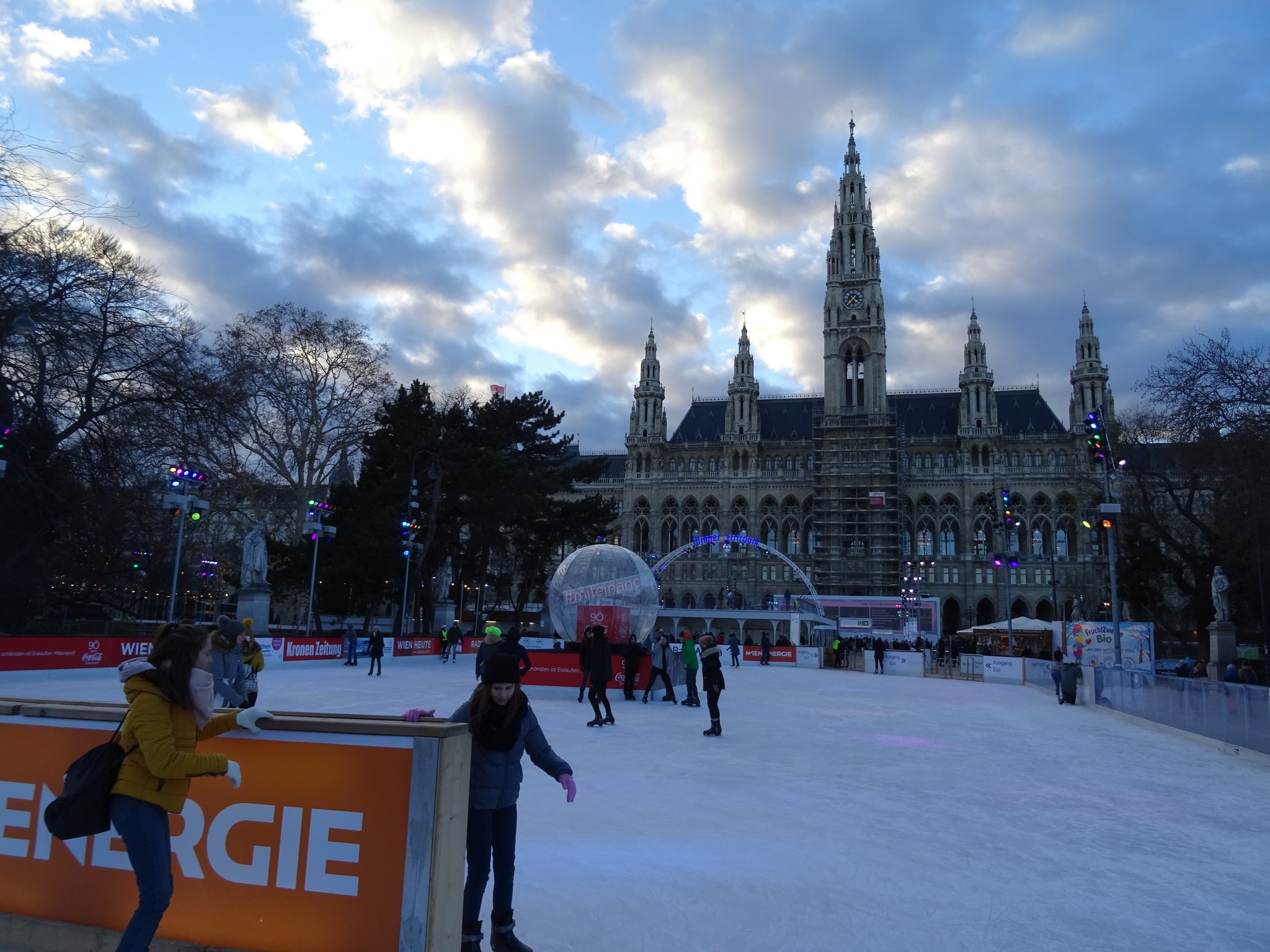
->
[726,576,736,610]
[964,605,978,629]
[161,466,210,623]
[303,499,338,638]
[1037,544,1058,621]
[1082,406,1126,671]
[132,551,152,619]
[923,634,926,649]
[201,560,219,621]
[475,584,480,637]
[895,560,935,641]
[937,635,939,660]
[988,484,1018,659]
[767,602,779,611]
[655,576,663,606]
[949,636,952,667]
[460,581,467,628]
[400,521,425,637]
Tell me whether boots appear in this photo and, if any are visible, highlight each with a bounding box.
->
[703,718,722,736]
[489,908,533,952]
[462,920,484,952]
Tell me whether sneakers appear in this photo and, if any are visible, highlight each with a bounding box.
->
[351,661,358,665]
[587,716,604,726]
[442,654,455,664]
[368,670,373,676]
[376,670,381,676]
[760,662,770,666]
[577,687,701,707]
[604,714,615,724]
[873,669,884,674]
[344,661,352,665]
[730,664,740,668]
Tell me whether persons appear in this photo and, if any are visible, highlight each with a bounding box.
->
[620,630,700,706]
[587,626,615,727]
[1051,662,1082,704]
[1175,650,1257,714]
[784,589,791,610]
[439,622,464,664]
[934,635,1064,667]
[475,625,532,683]
[43,624,276,952]
[577,626,601,704]
[402,639,576,952]
[718,587,733,609]
[832,634,932,674]
[698,635,725,736]
[242,521,272,587]
[343,622,385,676]
[1070,595,1084,621]
[704,630,797,668]
[181,615,264,708]
[1210,565,1231,622]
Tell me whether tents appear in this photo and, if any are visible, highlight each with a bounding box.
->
[956,616,1059,638]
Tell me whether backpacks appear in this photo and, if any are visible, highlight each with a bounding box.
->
[45,708,140,840]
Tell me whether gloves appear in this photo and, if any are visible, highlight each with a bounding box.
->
[236,706,274,733]
[560,773,576,803]
[225,759,241,788]
[402,709,435,721]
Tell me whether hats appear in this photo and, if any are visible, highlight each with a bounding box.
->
[698,635,714,649]
[375,626,381,631]
[481,626,521,687]
[453,622,458,626]
[218,615,245,641]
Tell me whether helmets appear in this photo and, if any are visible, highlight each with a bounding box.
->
[441,625,446,629]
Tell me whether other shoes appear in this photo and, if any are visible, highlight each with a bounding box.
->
[1056,694,1069,704]
[834,663,848,668]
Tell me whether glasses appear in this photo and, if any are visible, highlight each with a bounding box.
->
[586,632,592,634]
